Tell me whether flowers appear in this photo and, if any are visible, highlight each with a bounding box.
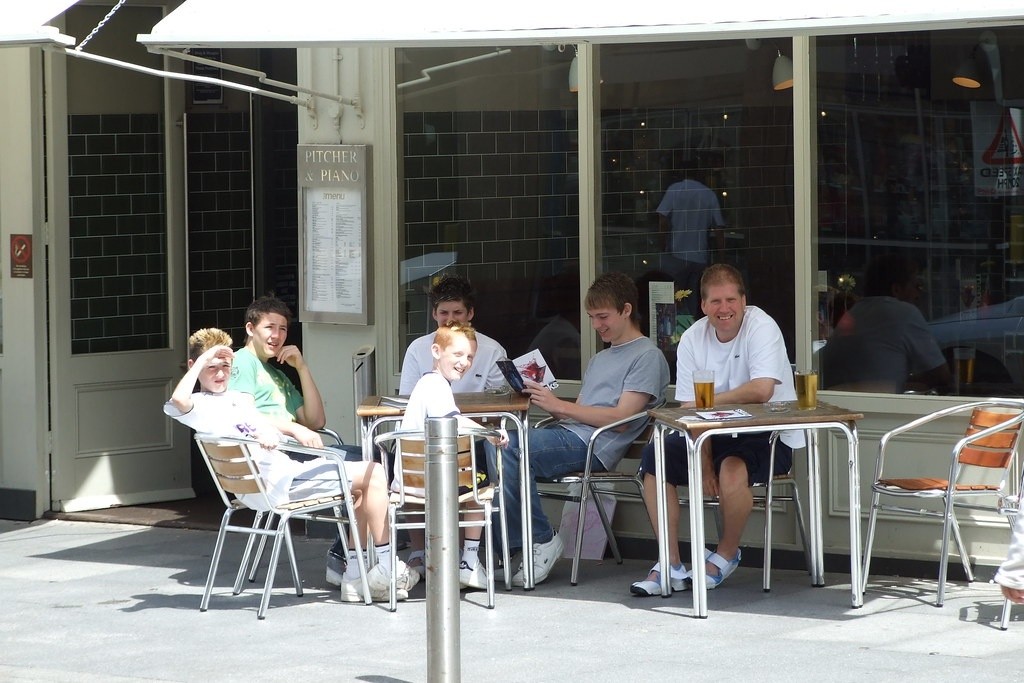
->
[837,274,856,313]
[675,288,693,315]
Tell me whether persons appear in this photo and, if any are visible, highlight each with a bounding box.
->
[390,324,509,589]
[629,265,806,598]
[227,297,384,586]
[398,275,507,569]
[485,271,670,586]
[656,164,725,262]
[529,261,954,397]
[164,328,420,602]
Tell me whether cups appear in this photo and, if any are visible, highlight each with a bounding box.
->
[794,370,818,410]
[692,369,715,410]
[953,347,976,383]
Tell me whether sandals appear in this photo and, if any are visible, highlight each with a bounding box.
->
[629,560,687,597]
[686,546,741,590]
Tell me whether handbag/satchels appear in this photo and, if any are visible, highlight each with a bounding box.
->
[557,482,616,560]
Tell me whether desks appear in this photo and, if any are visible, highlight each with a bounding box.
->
[355,392,532,604]
[644,400,862,620]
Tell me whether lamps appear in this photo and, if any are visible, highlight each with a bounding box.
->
[772,42,793,91]
[570,50,603,93]
[953,64,989,88]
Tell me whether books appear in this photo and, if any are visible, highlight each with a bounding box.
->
[696,409,755,420]
[285,435,347,460]
[496,348,558,396]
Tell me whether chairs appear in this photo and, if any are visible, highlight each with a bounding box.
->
[863,396,1024,632]
[195,397,800,620]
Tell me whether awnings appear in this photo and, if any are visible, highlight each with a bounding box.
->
[0,0,1024,117]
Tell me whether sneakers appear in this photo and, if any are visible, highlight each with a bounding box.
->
[459,560,496,590]
[368,559,420,591]
[407,552,425,579]
[340,572,408,601]
[512,526,565,586]
[325,550,348,587]
[493,552,522,582]
